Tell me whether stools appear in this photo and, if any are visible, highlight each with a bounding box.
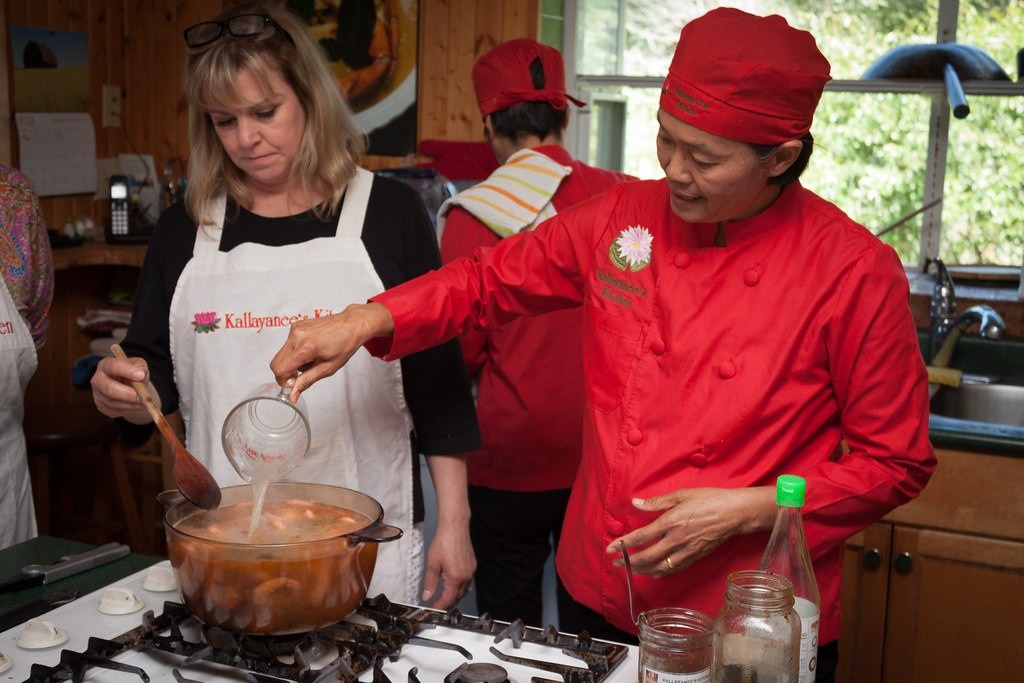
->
[24,403,113,535]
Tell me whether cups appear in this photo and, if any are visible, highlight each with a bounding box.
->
[221,368,311,479]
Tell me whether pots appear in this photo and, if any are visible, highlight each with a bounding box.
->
[154,480,405,636]
[861,42,1011,119]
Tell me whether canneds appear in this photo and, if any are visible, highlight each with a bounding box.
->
[635,570,802,683]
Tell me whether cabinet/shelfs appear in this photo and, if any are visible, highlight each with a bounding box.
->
[838,442,1024,683]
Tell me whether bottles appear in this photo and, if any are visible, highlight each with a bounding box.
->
[710,569,803,683]
[757,474,821,683]
[635,607,717,683]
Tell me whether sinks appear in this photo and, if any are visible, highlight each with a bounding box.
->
[926,374,1024,445]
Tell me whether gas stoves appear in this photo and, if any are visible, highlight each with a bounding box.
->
[1,554,645,683]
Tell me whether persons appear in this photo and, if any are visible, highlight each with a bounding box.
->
[433,37,649,646]
[270,7,938,683]
[0,159,57,549]
[91,11,485,613]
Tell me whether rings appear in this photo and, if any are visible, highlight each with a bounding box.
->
[666,556,679,570]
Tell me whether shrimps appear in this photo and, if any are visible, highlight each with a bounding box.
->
[180,499,358,610]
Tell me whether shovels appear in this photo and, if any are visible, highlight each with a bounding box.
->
[110,343,222,511]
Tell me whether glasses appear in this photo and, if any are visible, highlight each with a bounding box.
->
[183,11,296,64]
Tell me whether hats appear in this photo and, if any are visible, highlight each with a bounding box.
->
[658,7,833,145]
[472,39,586,121]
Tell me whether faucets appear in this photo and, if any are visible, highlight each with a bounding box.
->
[926,254,1007,370]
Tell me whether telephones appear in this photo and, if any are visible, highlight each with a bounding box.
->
[103,174,152,245]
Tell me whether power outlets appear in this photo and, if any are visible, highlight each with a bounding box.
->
[102,85,121,127]
